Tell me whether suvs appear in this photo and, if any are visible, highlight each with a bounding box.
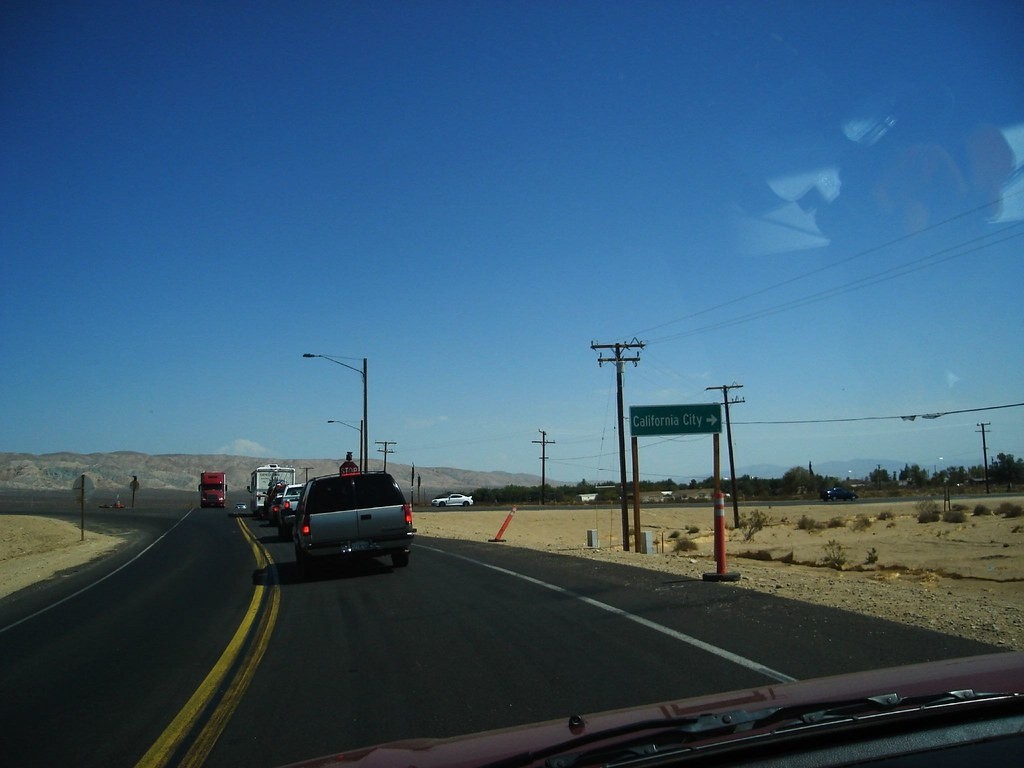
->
[281,470,417,572]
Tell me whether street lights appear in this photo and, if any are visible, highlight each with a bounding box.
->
[302,353,369,472]
[328,420,363,474]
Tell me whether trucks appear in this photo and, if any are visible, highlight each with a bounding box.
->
[247,464,296,513]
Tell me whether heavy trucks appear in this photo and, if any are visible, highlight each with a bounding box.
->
[197,472,228,508]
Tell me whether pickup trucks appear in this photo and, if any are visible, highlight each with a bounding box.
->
[277,483,309,541]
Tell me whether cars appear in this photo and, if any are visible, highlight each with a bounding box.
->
[236,502,247,509]
[261,482,286,520]
[432,493,473,507]
[821,488,859,501]
